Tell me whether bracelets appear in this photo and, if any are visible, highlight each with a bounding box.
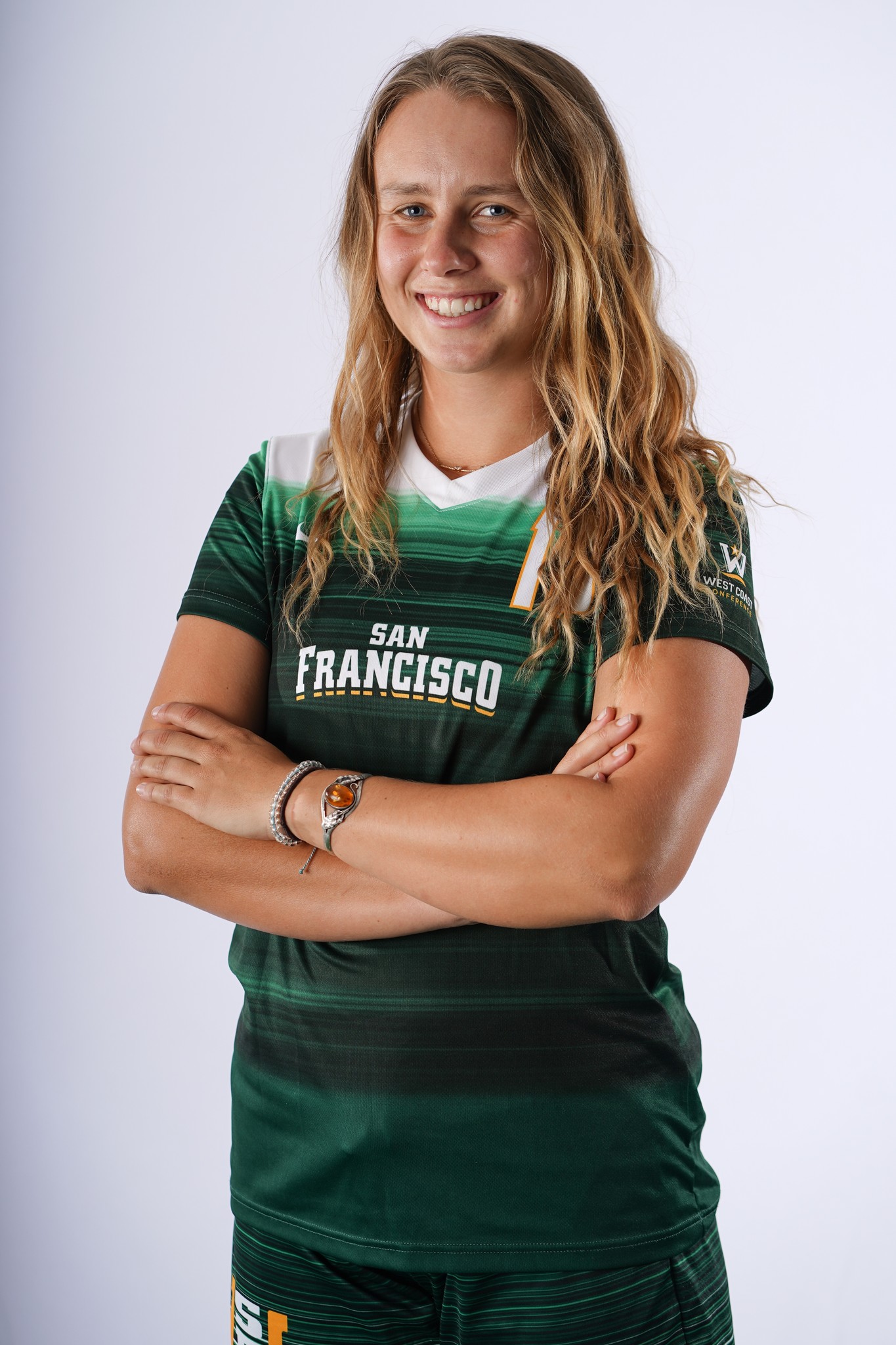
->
[316,771,373,853]
[268,757,323,849]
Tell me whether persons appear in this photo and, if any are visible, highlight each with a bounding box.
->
[122,33,777,1345]
